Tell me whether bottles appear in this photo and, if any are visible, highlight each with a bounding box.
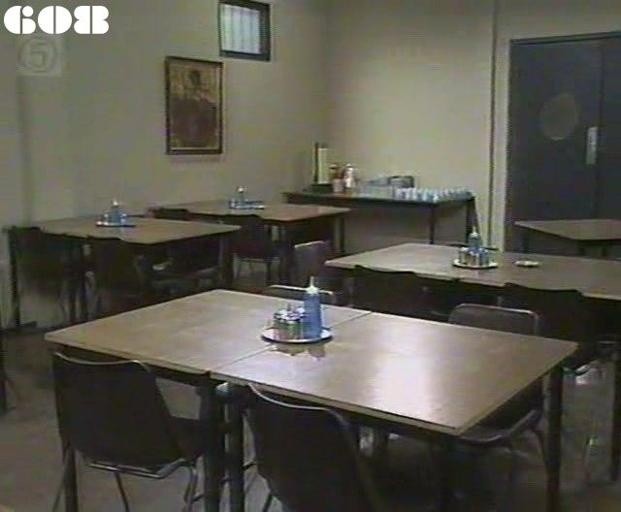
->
[225,185,264,211]
[258,273,331,343]
[92,197,127,228]
[326,163,357,194]
[582,433,602,489]
[450,225,499,268]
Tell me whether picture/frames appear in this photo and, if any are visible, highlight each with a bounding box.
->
[164,56,223,155]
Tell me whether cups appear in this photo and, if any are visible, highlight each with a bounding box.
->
[393,184,470,202]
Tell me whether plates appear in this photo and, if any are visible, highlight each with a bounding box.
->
[511,260,543,266]
